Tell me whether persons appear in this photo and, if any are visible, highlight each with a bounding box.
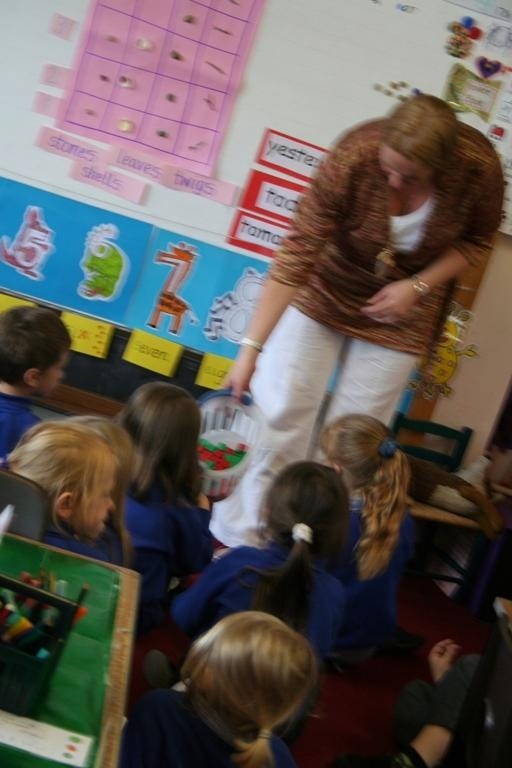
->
[391,637,481,767]
[170,461,348,680]
[118,610,320,767]
[113,382,213,632]
[0,419,120,567]
[209,94,503,564]
[0,304,71,457]
[68,414,135,569]
[317,412,415,673]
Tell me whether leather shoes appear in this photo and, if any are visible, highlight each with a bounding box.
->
[377,625,425,652]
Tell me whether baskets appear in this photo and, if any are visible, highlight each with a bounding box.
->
[197,391,265,501]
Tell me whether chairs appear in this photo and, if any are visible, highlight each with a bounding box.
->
[386,412,486,624]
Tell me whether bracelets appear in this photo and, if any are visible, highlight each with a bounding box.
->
[411,273,431,298]
[238,335,262,352]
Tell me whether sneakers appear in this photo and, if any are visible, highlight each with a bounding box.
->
[144,649,174,688]
